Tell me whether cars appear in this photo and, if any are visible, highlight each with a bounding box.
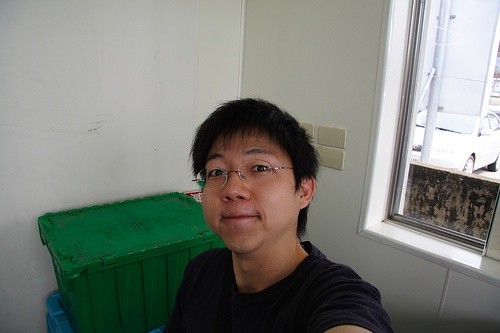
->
[477,82,499,116]
[409,105,499,177]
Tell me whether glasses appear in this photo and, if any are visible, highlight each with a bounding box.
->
[192,159,294,191]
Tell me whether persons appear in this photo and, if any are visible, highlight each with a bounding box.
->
[162,97,394,333]
